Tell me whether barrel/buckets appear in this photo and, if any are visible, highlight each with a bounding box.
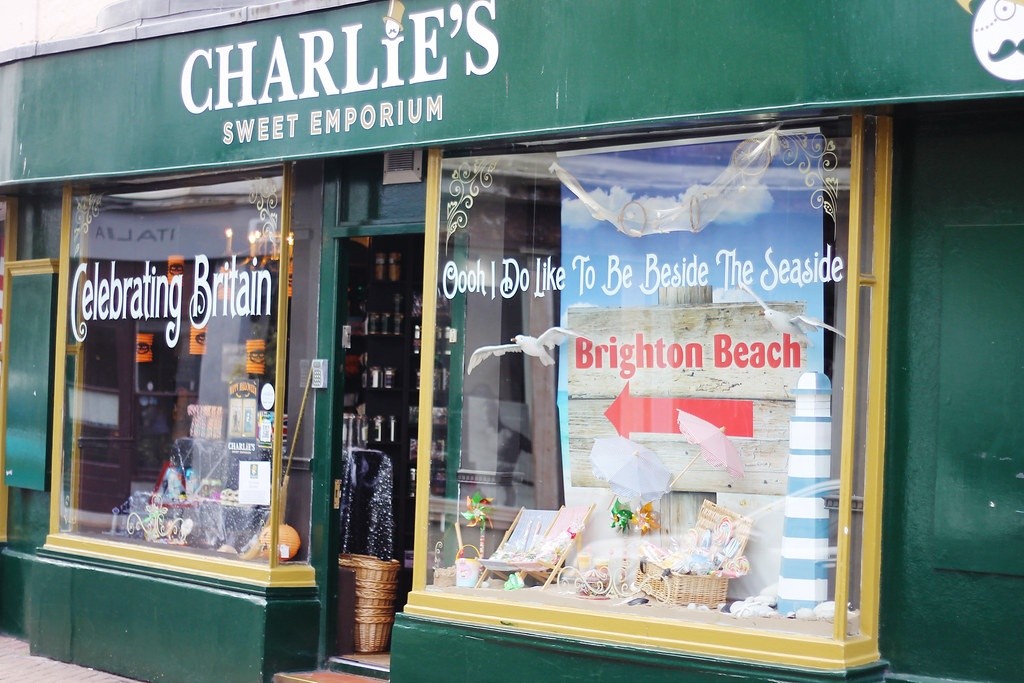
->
[455,545,482,586]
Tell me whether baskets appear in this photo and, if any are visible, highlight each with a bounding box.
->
[338,553,401,652]
[634,555,728,611]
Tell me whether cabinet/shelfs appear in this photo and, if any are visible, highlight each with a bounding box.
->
[139,236,454,571]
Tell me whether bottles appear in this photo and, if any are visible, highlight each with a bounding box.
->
[185,469,199,496]
[211,479,221,499]
[202,478,211,497]
[258,412,271,446]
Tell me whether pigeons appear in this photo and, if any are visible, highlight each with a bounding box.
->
[735,281,846,350]
[467,326,594,374]
